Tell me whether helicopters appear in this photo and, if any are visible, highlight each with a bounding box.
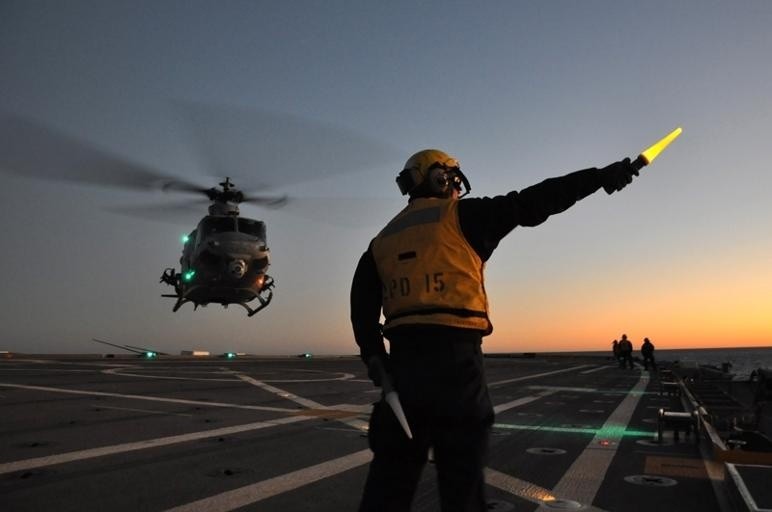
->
[4,117,403,317]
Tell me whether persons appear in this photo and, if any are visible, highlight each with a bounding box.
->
[640,337,656,371]
[618,333,634,371]
[611,339,620,363]
[350,146,637,511]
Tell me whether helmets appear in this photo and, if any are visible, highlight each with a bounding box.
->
[395,149,458,198]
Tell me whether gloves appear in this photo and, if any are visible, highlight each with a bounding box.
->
[596,158,639,192]
[367,356,390,386]
